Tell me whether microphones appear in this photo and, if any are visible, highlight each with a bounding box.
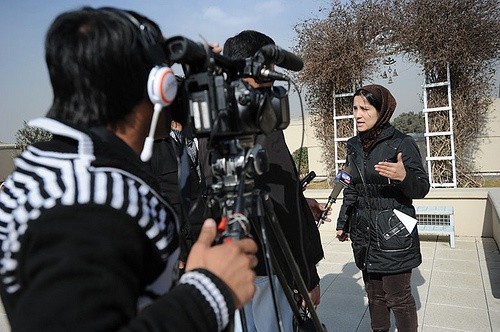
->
[300,171,316,188]
[141,106,163,162]
[317,166,353,228]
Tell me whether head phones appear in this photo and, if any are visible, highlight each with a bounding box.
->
[99,6,177,106]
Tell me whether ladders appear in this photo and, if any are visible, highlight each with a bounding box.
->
[422,49,458,189]
[332,71,358,177]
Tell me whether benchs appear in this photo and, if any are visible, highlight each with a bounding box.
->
[413,206,456,249]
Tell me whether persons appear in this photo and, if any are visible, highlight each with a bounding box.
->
[336,85,431,332]
[0,6,257,332]
[220,31,324,332]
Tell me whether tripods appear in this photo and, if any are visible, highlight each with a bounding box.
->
[199,136,326,332]
